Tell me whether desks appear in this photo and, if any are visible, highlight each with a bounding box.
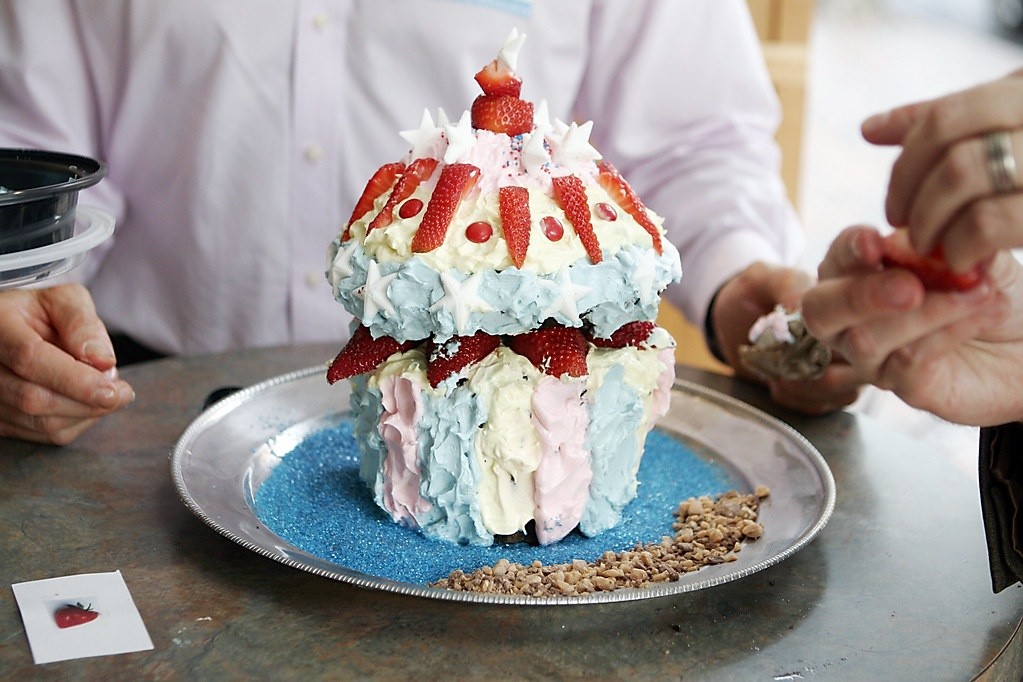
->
[0,342,1023,682]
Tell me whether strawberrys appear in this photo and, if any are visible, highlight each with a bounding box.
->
[880,229,988,293]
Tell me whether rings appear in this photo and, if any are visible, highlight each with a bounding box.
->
[982,131,1017,194]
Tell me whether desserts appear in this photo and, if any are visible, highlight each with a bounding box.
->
[325,29,686,549]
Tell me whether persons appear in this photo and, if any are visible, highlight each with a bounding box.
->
[802,69,1023,594]
[0,0,862,446]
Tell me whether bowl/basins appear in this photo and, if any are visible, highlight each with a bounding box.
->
[0,148,108,281]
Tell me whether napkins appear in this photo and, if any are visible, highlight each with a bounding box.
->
[12,569,154,667]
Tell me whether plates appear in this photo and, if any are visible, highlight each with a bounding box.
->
[0,205,116,292]
[171,362,836,607]
